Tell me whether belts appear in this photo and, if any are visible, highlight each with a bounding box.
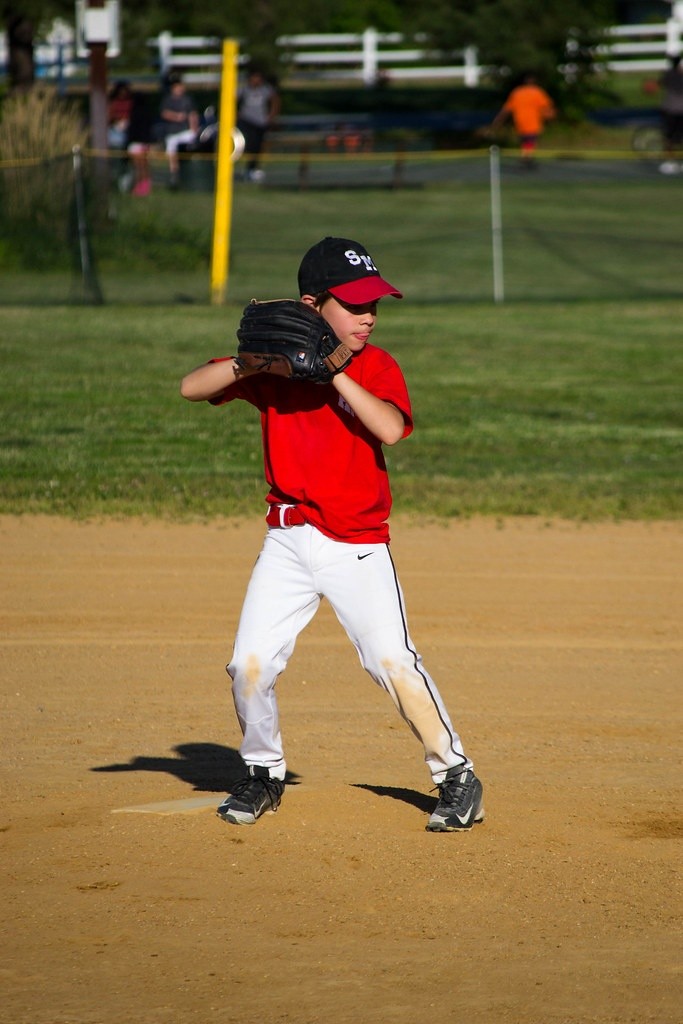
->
[266,505,311,529]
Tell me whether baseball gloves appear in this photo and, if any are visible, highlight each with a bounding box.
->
[236,297,354,384]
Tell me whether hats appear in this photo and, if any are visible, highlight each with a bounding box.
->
[298,237,403,305]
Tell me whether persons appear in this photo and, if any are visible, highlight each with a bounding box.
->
[489,69,555,171]
[83,36,279,197]
[179,233,485,834]
[650,54,683,178]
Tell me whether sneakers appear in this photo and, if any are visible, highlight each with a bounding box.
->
[217,764,286,826]
[427,768,485,832]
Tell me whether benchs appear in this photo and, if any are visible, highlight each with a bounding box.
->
[259,112,414,189]
